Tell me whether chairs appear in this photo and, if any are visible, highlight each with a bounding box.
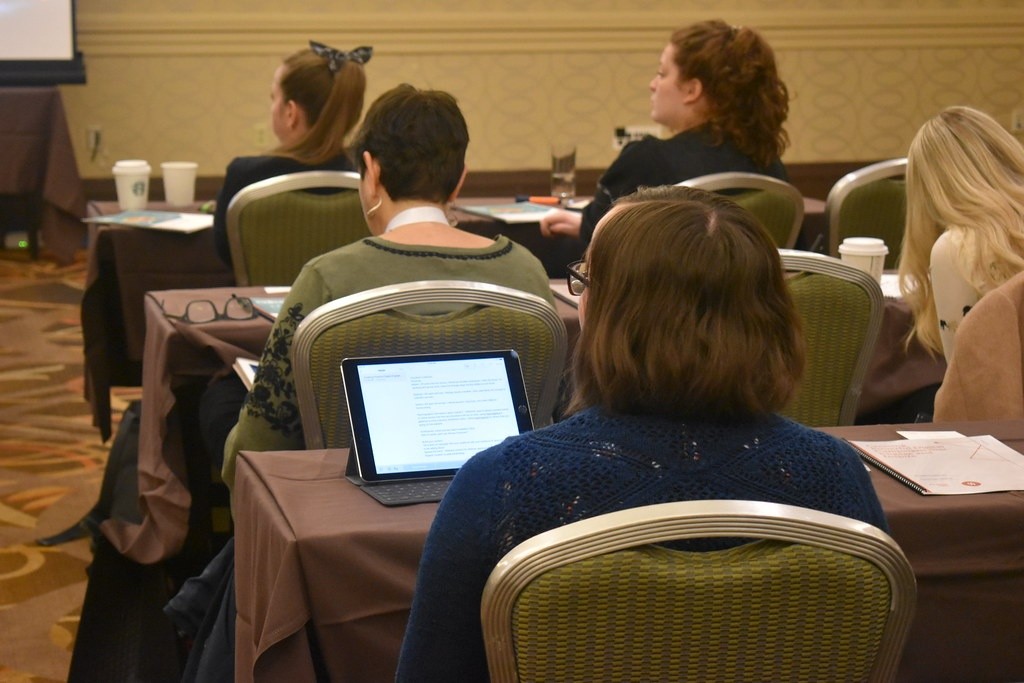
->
[770,246,888,431]
[669,173,806,256]
[228,168,373,289]
[479,502,911,683]
[291,280,571,452]
[825,157,910,272]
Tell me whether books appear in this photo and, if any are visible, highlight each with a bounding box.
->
[84,210,213,233]
[251,297,284,320]
[460,201,581,223]
[841,435,1024,495]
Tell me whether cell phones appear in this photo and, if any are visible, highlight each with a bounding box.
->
[250,297,283,322]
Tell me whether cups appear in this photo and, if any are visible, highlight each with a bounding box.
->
[112,160,150,210]
[160,160,197,207]
[552,146,575,200]
[838,237,889,288]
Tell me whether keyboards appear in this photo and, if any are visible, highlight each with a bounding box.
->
[361,480,451,506]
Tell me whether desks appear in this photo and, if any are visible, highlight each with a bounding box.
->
[77,192,1024,683]
[1,84,92,259]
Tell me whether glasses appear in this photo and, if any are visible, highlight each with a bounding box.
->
[159,293,258,324]
[565,259,589,296]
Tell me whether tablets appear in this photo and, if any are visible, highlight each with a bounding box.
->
[340,348,534,483]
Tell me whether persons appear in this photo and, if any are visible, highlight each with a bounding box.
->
[395,186,890,683]
[214,41,375,282]
[899,108,1024,365]
[540,19,790,257]
[202,83,559,513]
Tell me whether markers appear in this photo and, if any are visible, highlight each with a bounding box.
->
[515,196,561,205]
